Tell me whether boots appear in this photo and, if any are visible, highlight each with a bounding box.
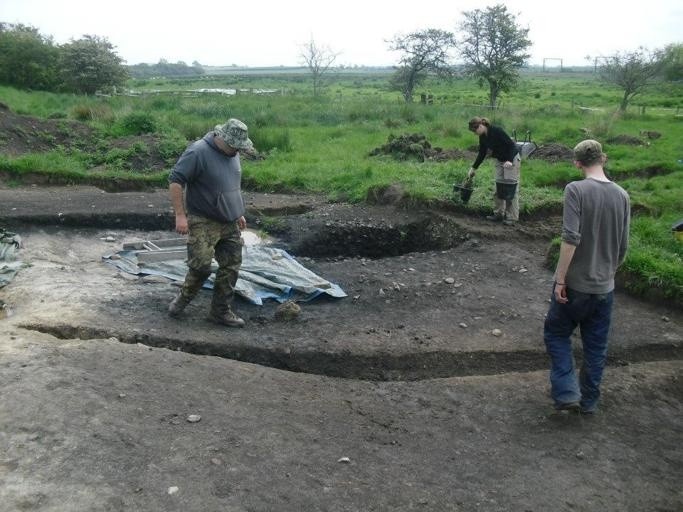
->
[502,218,517,226]
[167,289,191,315]
[206,305,245,329]
[486,212,505,222]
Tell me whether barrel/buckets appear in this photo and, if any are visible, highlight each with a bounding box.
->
[453,183,475,205]
[495,167,519,200]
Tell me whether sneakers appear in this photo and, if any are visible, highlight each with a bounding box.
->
[550,398,581,410]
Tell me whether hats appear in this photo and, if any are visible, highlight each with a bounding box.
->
[214,117,253,149]
[574,140,602,162]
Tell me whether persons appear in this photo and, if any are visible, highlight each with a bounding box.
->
[168,118,253,327]
[543,139,630,414]
[468,117,521,226]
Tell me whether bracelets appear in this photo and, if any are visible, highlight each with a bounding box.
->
[556,282,567,285]
